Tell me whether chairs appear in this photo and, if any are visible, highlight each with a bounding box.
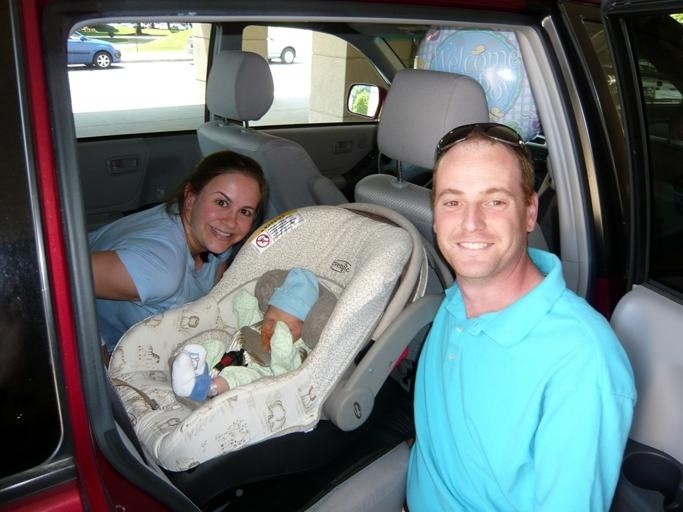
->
[299,281,683,512]
[106,202,412,469]
[197,48,347,217]
[358,66,547,266]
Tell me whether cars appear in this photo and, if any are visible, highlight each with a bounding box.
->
[185,25,300,67]
[66,30,120,69]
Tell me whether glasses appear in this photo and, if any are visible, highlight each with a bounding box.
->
[435,122,525,160]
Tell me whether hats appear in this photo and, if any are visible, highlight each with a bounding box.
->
[268,267,320,323]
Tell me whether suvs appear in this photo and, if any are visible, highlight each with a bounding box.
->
[0,0,682,510]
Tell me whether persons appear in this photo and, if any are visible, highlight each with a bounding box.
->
[86,150,270,357]
[401,125,638,512]
[172,268,319,400]
